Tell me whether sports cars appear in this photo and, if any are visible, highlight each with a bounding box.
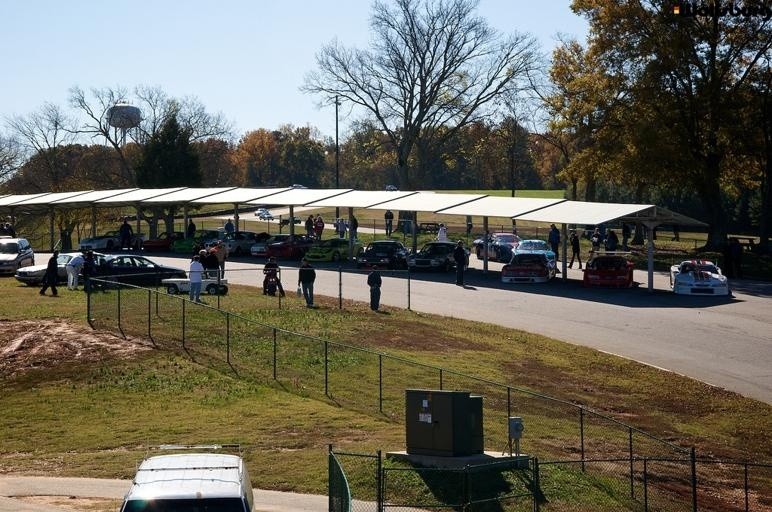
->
[79,228,146,253]
[581,249,635,289]
[669,258,729,296]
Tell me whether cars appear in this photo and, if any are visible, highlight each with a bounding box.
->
[472,233,522,264]
[0,236,13,239]
[406,240,472,274]
[304,237,365,263]
[385,185,398,190]
[288,183,309,189]
[140,230,316,260]
[78,254,187,289]
[357,239,412,269]
[288,216,301,224]
[14,252,108,286]
[253,208,268,216]
[259,211,272,220]
[730,236,772,280]
[511,239,557,267]
[501,250,557,283]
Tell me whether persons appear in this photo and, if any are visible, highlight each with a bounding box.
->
[120,220,133,252]
[0,224,14,239]
[670,223,680,242]
[368,265,382,310]
[438,223,446,242]
[549,224,560,262]
[584,223,633,261]
[188,219,196,238]
[65,249,96,293]
[199,239,226,279]
[39,249,60,297]
[298,258,316,307]
[188,255,204,303]
[453,240,465,285]
[263,257,286,297]
[567,232,583,269]
[225,219,233,234]
[305,214,324,240]
[384,210,394,236]
[336,215,358,239]
[714,236,745,278]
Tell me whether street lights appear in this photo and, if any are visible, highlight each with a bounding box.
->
[332,93,343,225]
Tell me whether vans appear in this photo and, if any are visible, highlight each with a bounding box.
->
[117,442,255,511]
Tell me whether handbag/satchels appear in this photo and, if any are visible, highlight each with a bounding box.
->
[296,286,301,297]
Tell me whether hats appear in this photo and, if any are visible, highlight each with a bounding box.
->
[199,249,207,255]
[209,248,218,254]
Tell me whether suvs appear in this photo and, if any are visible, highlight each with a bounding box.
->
[161,268,229,296]
[0,238,34,275]
